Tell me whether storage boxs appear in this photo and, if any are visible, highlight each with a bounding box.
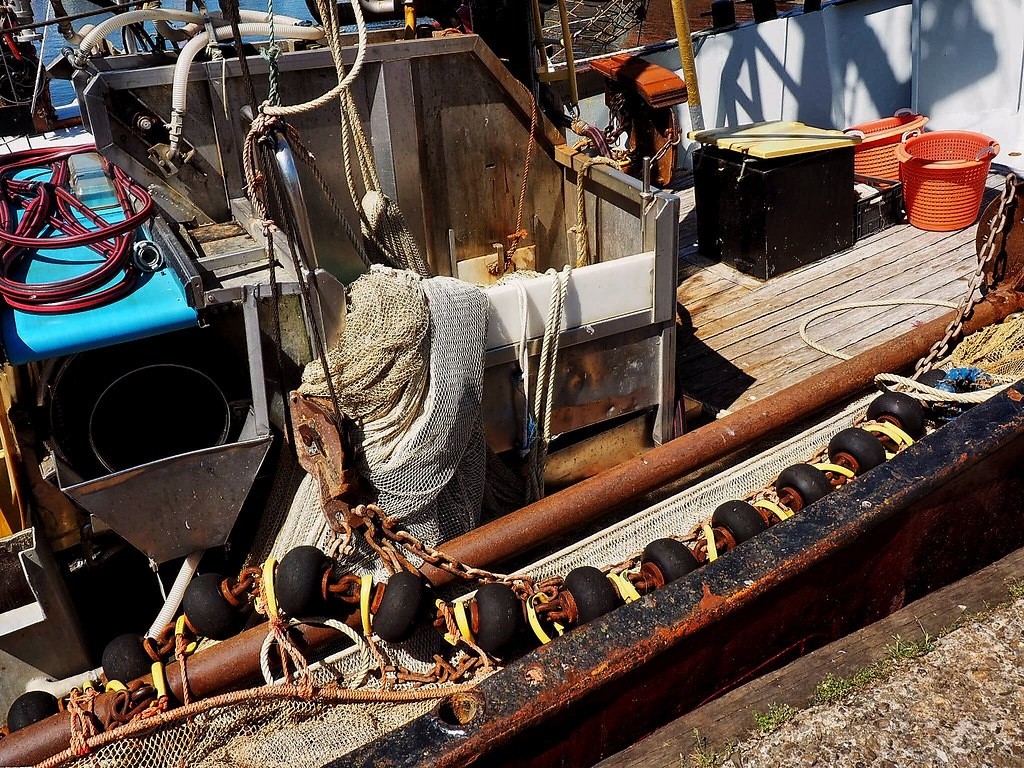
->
[854,172,905,243]
[687,119,860,280]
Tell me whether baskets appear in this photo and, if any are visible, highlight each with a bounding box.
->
[895,128,1000,232]
[842,108,930,188]
